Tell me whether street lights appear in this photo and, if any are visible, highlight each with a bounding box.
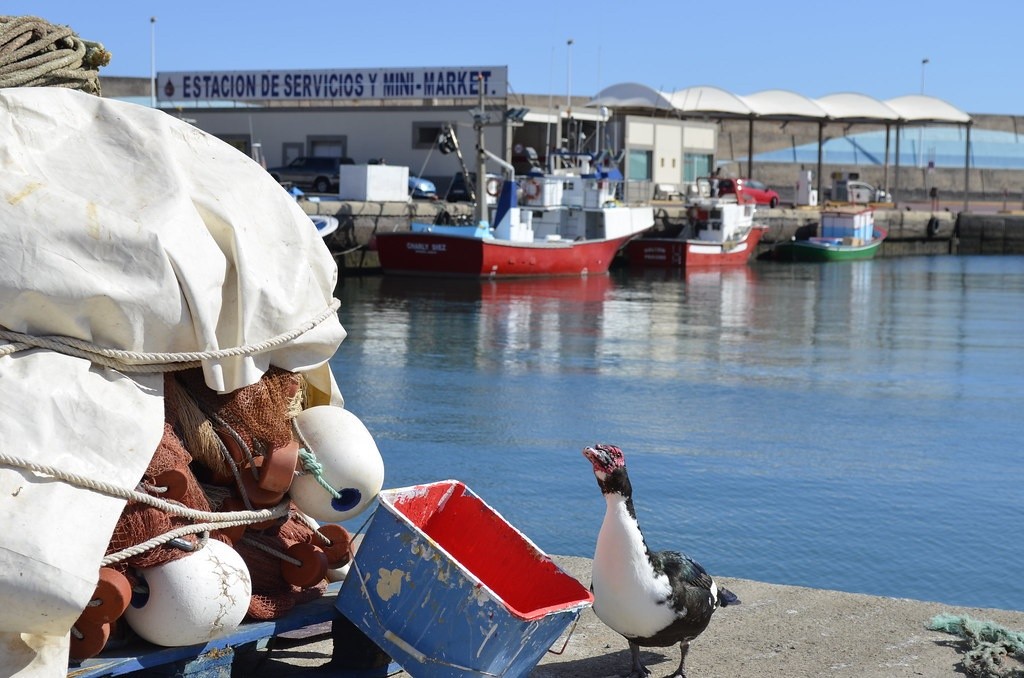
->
[563,38,574,172]
[917,58,930,168]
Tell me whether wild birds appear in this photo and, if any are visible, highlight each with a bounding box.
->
[583,443,737,678]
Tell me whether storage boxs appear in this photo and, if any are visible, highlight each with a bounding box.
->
[340,163,409,204]
[335,478,595,678]
[844,238,865,247]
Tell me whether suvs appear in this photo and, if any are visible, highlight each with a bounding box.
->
[266,155,356,192]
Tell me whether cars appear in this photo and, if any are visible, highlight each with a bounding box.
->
[408,176,437,200]
[718,177,781,208]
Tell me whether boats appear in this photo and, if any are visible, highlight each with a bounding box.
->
[774,203,887,259]
[375,71,658,278]
[306,215,339,247]
[629,200,769,266]
